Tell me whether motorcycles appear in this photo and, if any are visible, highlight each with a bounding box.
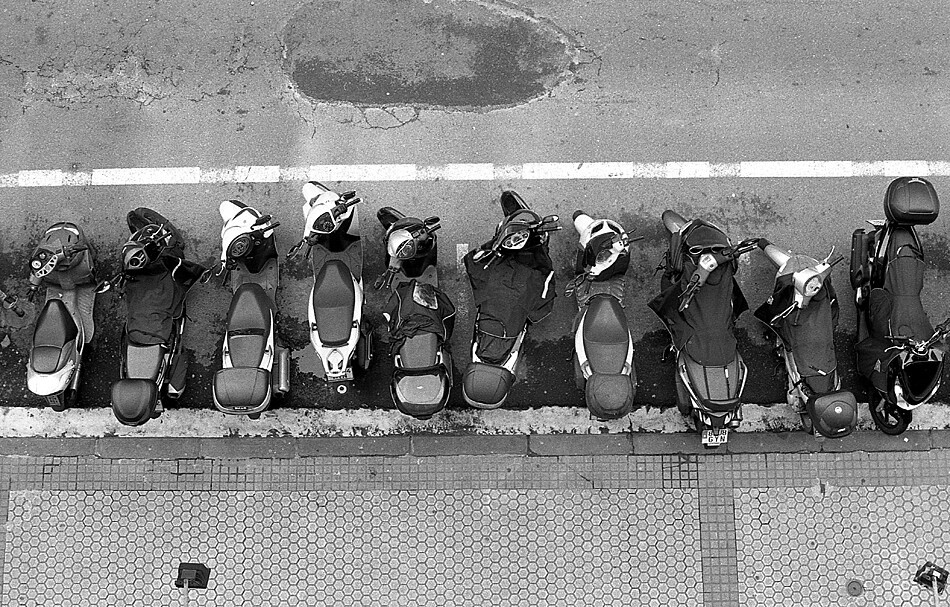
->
[287,181,375,401]
[462,190,563,410]
[563,209,646,421]
[199,199,292,420]
[755,237,859,443]
[374,207,457,419]
[95,205,204,427]
[26,221,100,413]
[647,210,761,451]
[850,176,950,436]
